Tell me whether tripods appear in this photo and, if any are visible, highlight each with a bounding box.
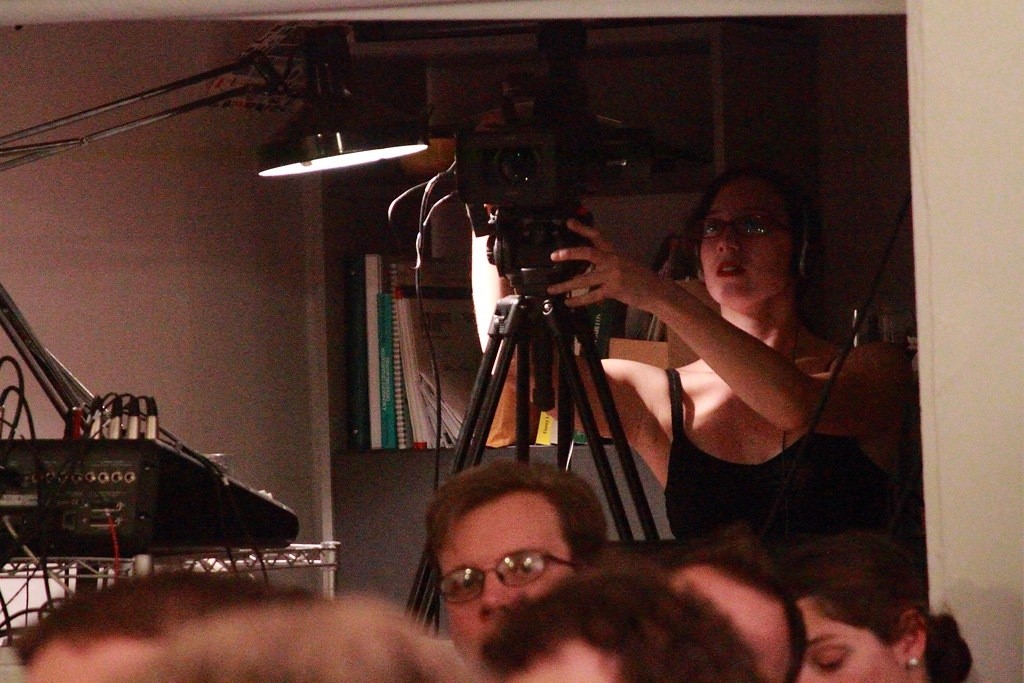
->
[402,262,658,631]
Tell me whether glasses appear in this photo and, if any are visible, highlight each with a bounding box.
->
[692,216,789,240]
[436,546,576,604]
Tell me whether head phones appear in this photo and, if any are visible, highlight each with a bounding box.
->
[694,200,817,281]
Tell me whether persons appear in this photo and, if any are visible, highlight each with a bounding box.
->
[467,168,912,541]
[9,460,971,683]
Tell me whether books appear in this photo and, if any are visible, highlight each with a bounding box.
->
[365,235,683,449]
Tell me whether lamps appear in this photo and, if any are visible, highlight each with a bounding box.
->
[0,22,432,441]
